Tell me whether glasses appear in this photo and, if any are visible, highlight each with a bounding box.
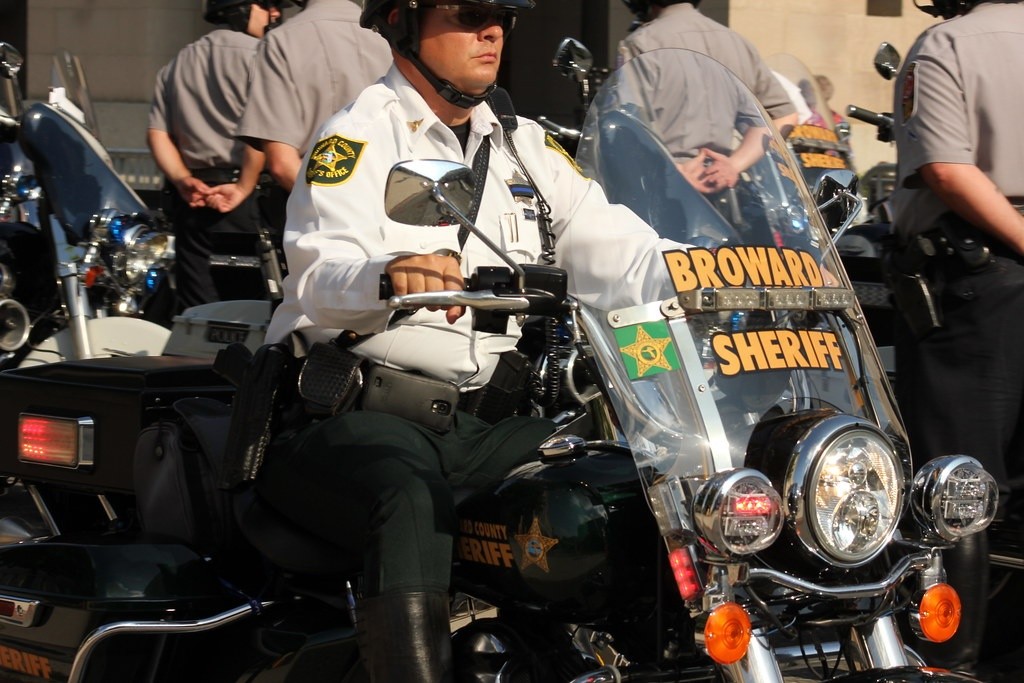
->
[428,1,519,32]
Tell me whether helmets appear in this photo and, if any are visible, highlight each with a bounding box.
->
[359,0,534,32]
[203,0,293,33]
[914,0,1024,19]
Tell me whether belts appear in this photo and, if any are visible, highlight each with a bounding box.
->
[190,171,277,183]
[920,227,1024,267]
[458,390,532,425]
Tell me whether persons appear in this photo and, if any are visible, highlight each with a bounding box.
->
[228,0,656,683]
[145,0,293,312]
[599,0,850,245]
[234,0,396,192]
[876,1,1023,676]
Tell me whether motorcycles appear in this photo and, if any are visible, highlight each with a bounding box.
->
[0,38,1024,683]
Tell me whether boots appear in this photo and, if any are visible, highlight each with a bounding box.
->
[343,590,451,683]
[916,529,988,676]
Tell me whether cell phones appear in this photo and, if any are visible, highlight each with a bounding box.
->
[361,366,460,432]
